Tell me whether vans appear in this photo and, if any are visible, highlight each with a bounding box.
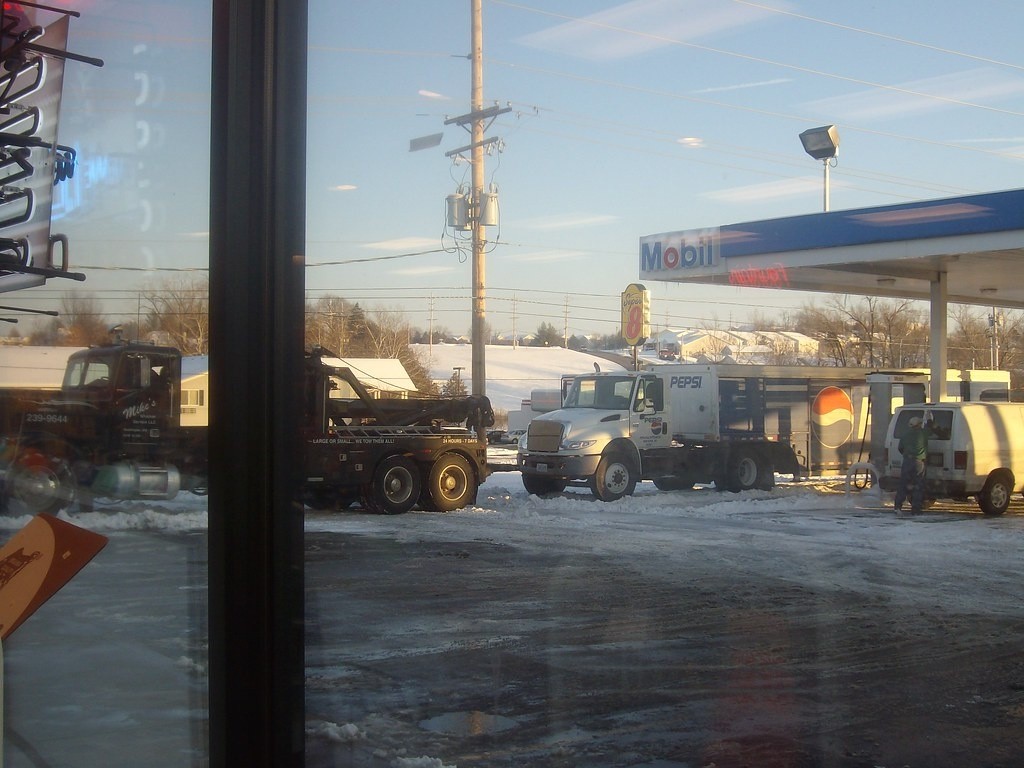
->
[882,396,1024,515]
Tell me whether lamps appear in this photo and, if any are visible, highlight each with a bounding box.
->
[798,125,841,160]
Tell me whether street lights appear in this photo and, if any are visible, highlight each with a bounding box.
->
[800,125,841,213]
[446,186,499,489]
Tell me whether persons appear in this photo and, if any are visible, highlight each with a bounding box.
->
[894,410,934,516]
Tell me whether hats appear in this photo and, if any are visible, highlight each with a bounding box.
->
[907,417,923,427]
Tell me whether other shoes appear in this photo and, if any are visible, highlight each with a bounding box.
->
[893,508,903,515]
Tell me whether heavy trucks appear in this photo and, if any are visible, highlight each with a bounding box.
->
[1,321,496,515]
[514,361,1011,505]
